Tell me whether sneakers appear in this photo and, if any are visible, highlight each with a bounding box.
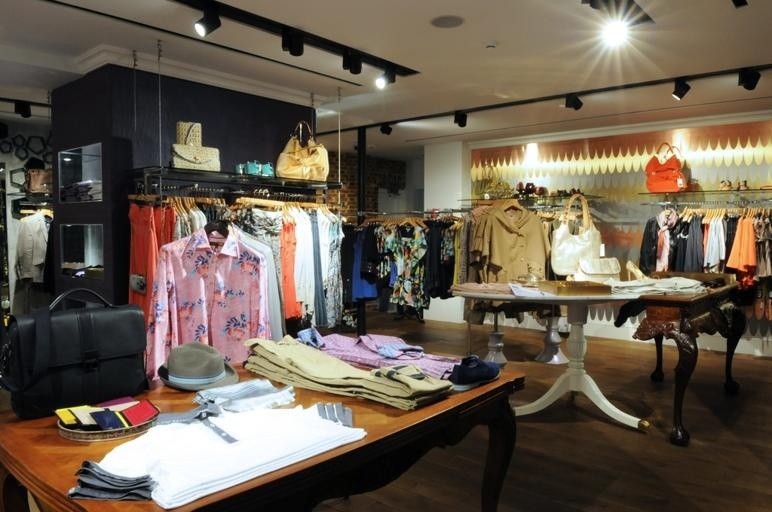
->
[446,356,501,393]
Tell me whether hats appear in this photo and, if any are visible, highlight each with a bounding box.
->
[154,339,241,395]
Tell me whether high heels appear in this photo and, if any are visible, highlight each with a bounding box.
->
[624,260,651,282]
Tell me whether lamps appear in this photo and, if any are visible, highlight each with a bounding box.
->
[194,2,396,90]
[380,65,762,137]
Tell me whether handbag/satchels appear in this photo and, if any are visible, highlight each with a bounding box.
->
[642,141,690,192]
[275,119,331,182]
[168,143,224,172]
[1,284,150,422]
[547,192,603,276]
[175,118,202,146]
[22,166,55,196]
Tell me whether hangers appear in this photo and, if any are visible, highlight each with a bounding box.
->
[19,207,53,222]
[346,197,590,238]
[128,184,347,246]
[648,200,772,226]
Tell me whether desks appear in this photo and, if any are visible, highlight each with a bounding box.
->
[454,280,679,431]
[0,345,526,512]
